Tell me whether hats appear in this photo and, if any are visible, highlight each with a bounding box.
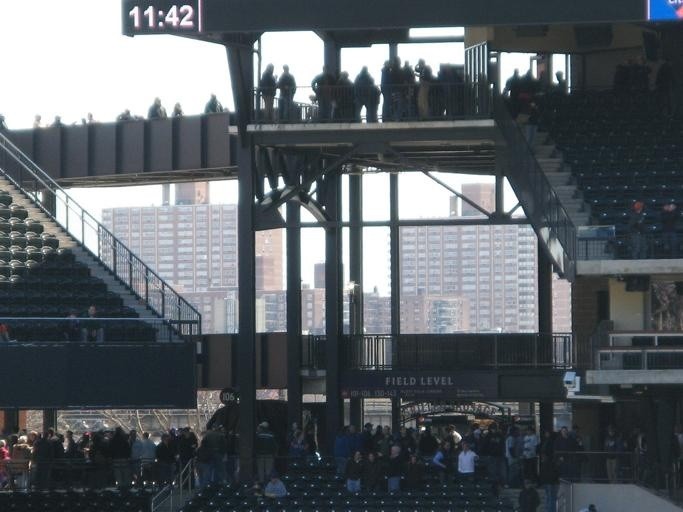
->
[635,201,644,210]
[259,421,270,428]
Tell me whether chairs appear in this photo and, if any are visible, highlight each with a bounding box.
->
[0,189,160,340]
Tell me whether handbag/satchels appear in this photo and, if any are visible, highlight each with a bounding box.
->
[508,445,525,459]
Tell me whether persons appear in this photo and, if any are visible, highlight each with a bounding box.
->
[204,94,221,112]
[277,64,296,118]
[170,102,187,116]
[55,307,88,345]
[624,199,652,260]
[146,97,168,118]
[259,64,279,118]
[308,64,569,124]
[86,112,98,123]
[115,108,133,120]
[48,114,68,128]
[657,198,683,259]
[80,304,104,342]
[32,115,43,128]
[1,409,681,511]
